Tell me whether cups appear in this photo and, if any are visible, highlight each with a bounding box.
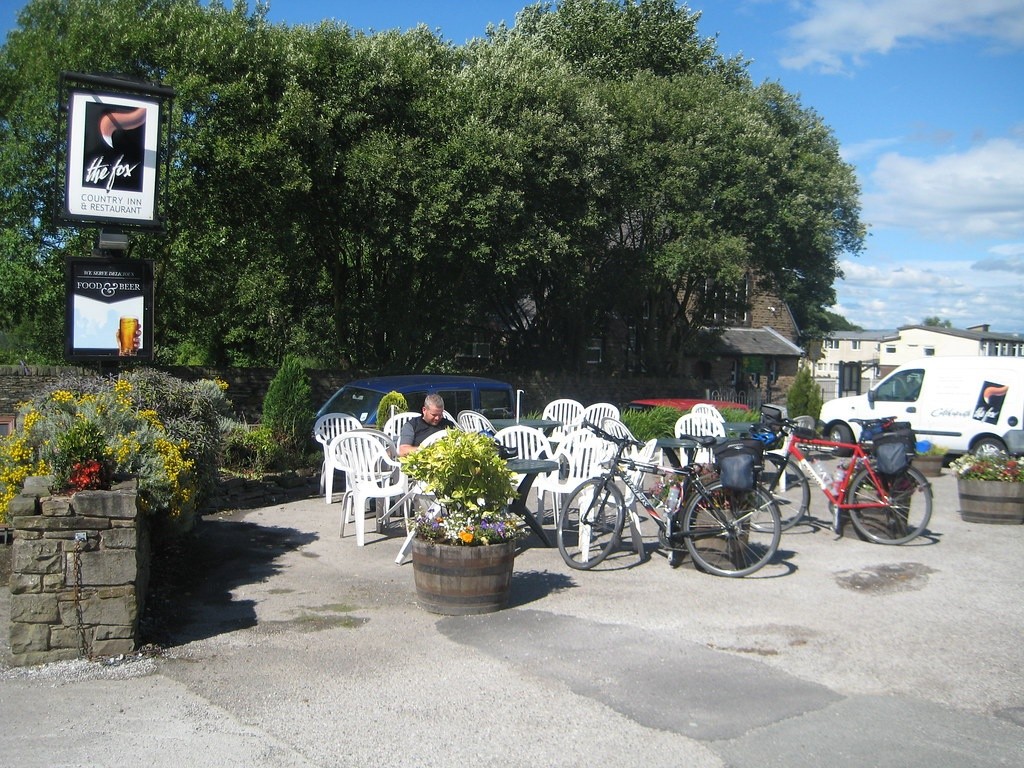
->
[119,315,139,357]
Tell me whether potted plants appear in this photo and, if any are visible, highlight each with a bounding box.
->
[957,478,1024,526]
[396,425,522,615]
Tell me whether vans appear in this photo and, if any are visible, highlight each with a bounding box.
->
[313,373,516,450]
[817,353,1024,461]
[626,399,750,415]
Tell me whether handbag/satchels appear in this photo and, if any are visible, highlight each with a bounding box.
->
[714,438,764,491]
[872,429,917,476]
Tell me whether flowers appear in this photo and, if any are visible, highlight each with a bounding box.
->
[949,453,1024,482]
[410,507,531,548]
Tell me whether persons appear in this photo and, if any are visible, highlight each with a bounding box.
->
[399,394,456,457]
[895,373,924,401]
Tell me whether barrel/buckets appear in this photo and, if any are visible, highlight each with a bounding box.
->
[914,440,932,454]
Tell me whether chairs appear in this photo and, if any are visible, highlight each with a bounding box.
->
[313,399,815,565]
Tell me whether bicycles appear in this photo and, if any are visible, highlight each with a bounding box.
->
[731,405,934,544]
[556,416,782,579]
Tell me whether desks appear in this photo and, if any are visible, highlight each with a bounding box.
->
[502,457,561,548]
[487,418,565,439]
[649,436,729,468]
[722,422,760,437]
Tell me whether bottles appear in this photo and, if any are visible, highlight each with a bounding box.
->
[812,458,834,488]
[831,465,846,495]
[665,485,681,513]
